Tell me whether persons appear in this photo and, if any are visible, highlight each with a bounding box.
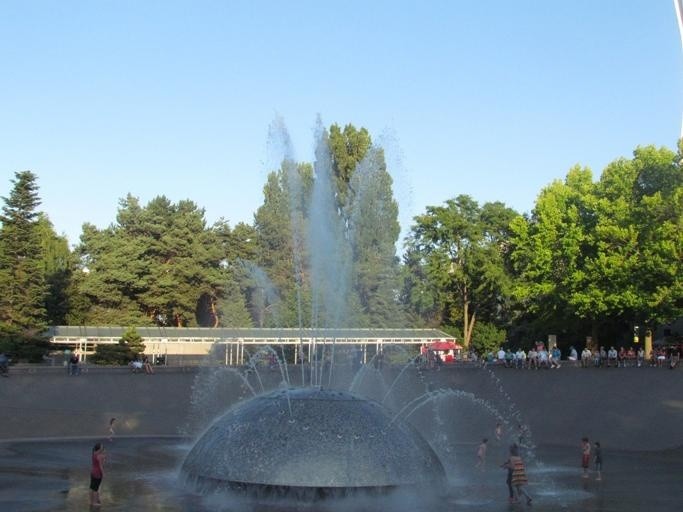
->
[86,442,106,506]
[518,423,531,444]
[502,444,533,506]
[580,437,591,479]
[475,437,489,467]
[493,423,503,443]
[415,350,443,382]
[128,356,155,376]
[499,447,521,502]
[593,441,602,481]
[567,345,577,361]
[1,353,9,376]
[453,341,562,371]
[109,416,120,435]
[63,346,78,373]
[581,344,683,369]
